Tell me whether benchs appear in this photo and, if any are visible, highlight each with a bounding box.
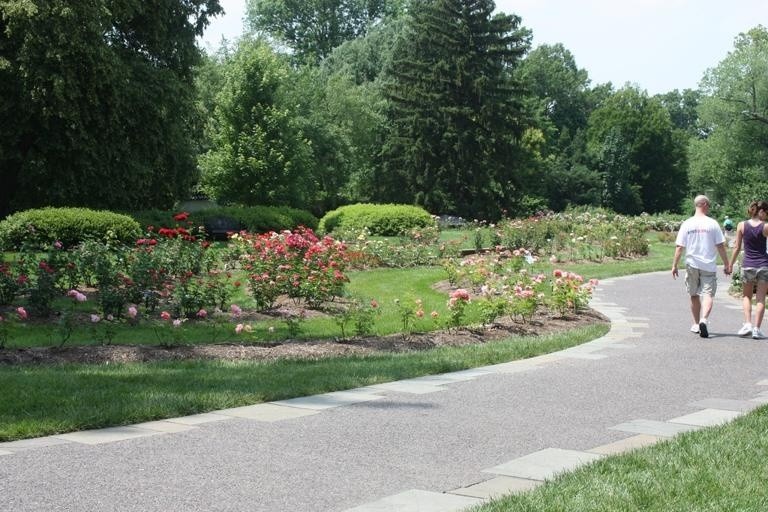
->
[460,248,494,256]
[210,216,238,243]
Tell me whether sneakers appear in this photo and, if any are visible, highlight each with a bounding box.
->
[698,317,709,339]
[752,327,768,340]
[736,324,753,337]
[690,322,700,333]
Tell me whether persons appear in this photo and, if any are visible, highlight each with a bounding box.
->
[671,194,730,338]
[723,215,734,237]
[730,200,768,339]
